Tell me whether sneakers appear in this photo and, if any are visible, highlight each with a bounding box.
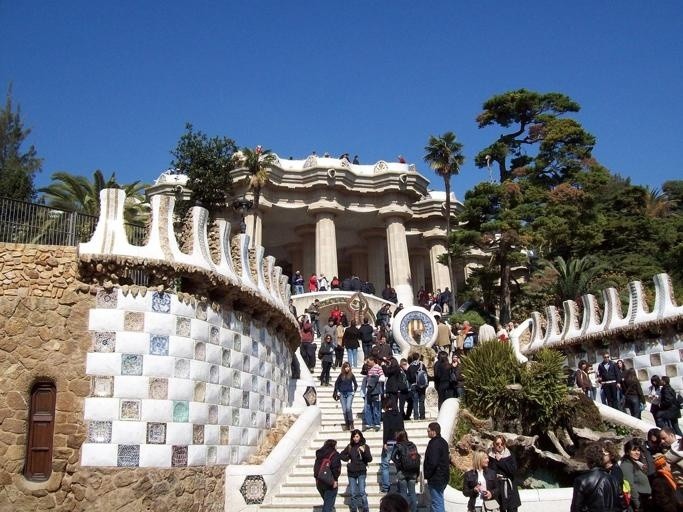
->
[346,422,354,431]
[401,413,426,420]
[320,379,329,386]
[365,423,381,431]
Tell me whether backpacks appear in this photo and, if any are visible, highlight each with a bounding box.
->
[464,336,474,350]
[672,391,683,409]
[417,371,428,388]
[398,443,420,473]
[332,279,340,288]
[398,367,410,392]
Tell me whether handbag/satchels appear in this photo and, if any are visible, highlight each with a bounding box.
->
[333,389,340,401]
[621,477,631,508]
[360,364,370,374]
[620,396,629,408]
[482,500,501,512]
[317,451,338,486]
[318,349,325,360]
[416,484,429,509]
[450,370,457,384]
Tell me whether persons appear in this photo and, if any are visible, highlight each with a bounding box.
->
[398,155,405,163]
[286,266,682,511]
[312,152,360,166]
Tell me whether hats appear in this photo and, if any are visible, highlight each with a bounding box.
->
[651,455,667,469]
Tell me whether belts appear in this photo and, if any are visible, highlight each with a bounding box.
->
[386,443,397,447]
